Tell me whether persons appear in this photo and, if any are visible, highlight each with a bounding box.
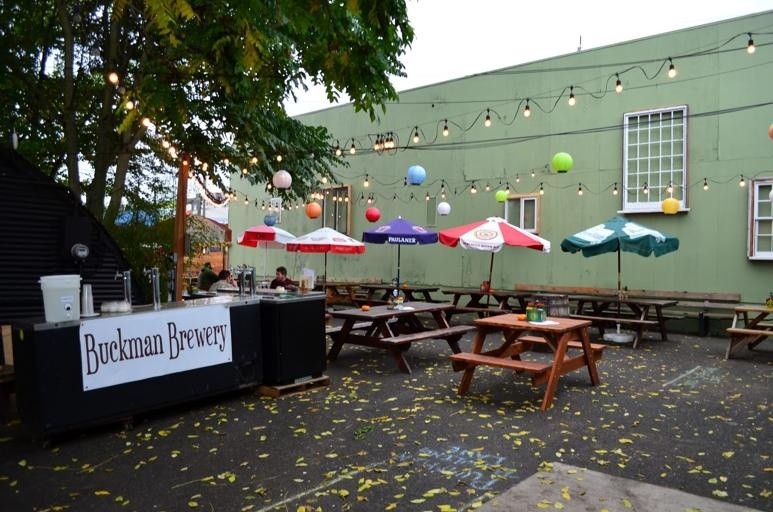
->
[198,263,219,290]
[229,272,238,288]
[271,266,293,291]
[209,269,240,291]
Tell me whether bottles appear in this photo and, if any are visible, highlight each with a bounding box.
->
[480,280,490,292]
[525,299,546,323]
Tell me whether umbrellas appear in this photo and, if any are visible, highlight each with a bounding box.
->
[146,215,232,246]
[362,218,439,299]
[237,225,297,280]
[286,226,367,294]
[438,216,551,317]
[560,215,679,334]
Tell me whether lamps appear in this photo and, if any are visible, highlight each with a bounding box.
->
[494,189,508,204]
[303,201,323,221]
[407,163,426,187]
[660,196,681,214]
[271,169,293,197]
[435,201,452,217]
[263,212,278,228]
[550,150,573,174]
[366,205,382,224]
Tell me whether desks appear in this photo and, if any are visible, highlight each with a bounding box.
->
[217,278,772,413]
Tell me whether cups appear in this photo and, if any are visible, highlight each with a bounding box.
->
[82,284,94,316]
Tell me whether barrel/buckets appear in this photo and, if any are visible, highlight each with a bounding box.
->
[38,275,83,323]
